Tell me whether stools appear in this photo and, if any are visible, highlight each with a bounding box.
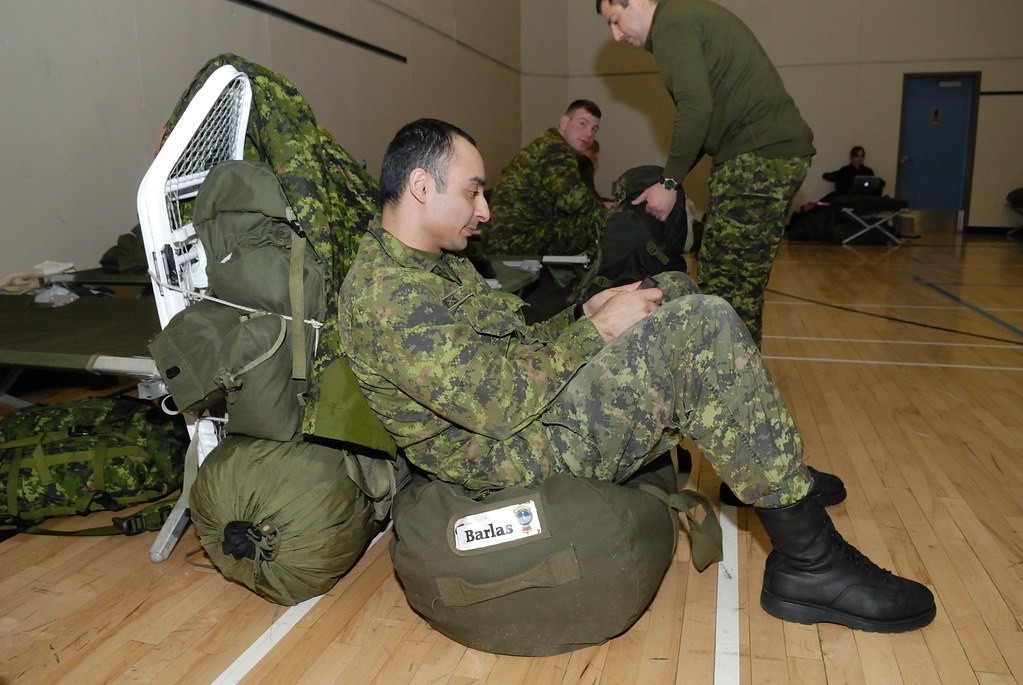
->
[831,197,911,245]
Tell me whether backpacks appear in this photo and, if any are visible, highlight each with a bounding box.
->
[596,166,688,285]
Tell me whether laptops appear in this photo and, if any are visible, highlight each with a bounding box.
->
[851,175,881,196]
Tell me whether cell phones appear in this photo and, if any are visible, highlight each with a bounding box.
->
[636,275,660,291]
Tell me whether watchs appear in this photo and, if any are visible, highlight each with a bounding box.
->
[660,177,680,191]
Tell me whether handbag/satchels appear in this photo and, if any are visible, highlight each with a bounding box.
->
[0,393,190,541]
[147,156,325,442]
[788,207,833,241]
[392,445,723,657]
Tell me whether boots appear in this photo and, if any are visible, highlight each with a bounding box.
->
[750,472,935,633]
[719,465,847,508]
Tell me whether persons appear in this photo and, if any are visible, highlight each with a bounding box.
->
[597,0,814,349]
[823,146,875,192]
[482,100,614,256]
[337,119,938,633]
